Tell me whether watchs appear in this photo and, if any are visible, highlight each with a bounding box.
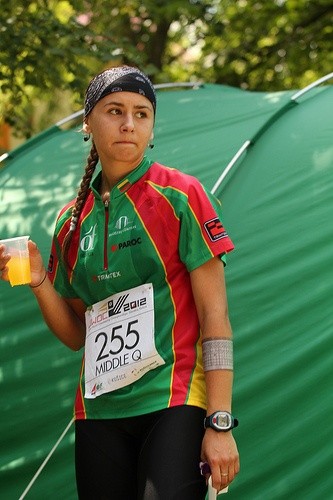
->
[203,409,238,432]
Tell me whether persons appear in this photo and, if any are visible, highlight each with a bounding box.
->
[0,60,243,500]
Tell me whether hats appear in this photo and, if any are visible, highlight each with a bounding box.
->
[84,65,158,113]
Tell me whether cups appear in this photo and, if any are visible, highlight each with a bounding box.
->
[0,235,32,287]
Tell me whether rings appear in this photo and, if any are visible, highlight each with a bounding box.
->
[221,473,229,476]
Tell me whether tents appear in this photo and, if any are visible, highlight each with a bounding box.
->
[0,75,332,499]
